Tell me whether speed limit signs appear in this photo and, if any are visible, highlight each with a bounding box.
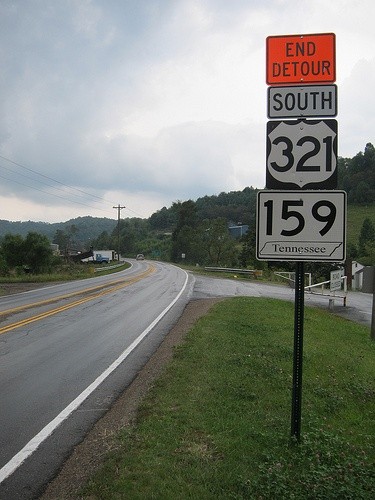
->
[255,118,347,265]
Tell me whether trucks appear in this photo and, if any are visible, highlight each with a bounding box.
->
[82,251,115,264]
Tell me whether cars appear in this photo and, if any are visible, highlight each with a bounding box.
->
[136,254,144,260]
